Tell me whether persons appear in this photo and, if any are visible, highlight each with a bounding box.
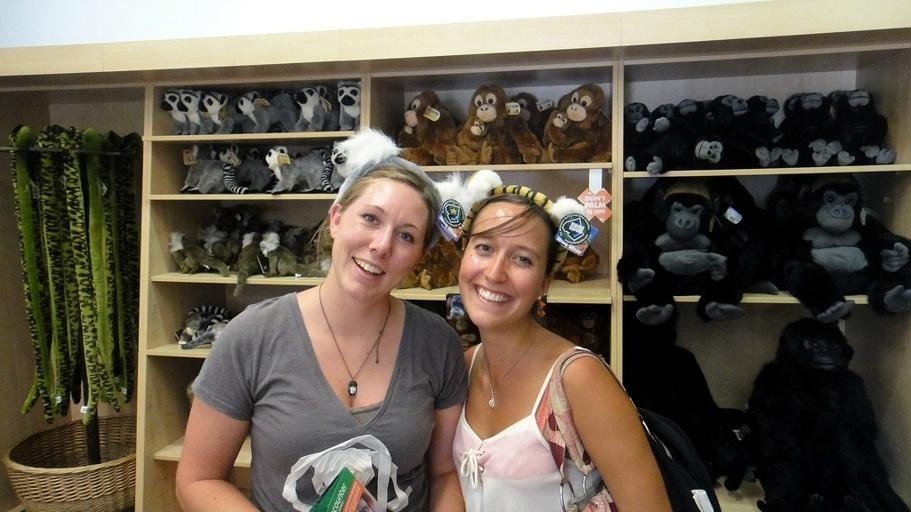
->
[450,192,675,512]
[174,164,468,512]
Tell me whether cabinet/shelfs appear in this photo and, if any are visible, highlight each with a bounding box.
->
[616,0,911,512]
[135,31,365,512]
[364,13,619,380]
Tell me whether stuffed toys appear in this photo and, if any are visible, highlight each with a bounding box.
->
[384,84,612,167]
[172,306,238,350]
[169,208,333,297]
[615,89,909,364]
[161,80,911,364]
[8,121,138,426]
[178,143,351,193]
[394,170,598,316]
[744,316,899,510]
[623,299,748,492]
[561,308,607,358]
[160,81,361,131]
[446,293,478,348]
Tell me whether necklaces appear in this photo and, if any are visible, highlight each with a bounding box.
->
[319,281,394,399]
[488,332,535,408]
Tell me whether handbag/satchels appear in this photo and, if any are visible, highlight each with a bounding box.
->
[548,347,724,512]
